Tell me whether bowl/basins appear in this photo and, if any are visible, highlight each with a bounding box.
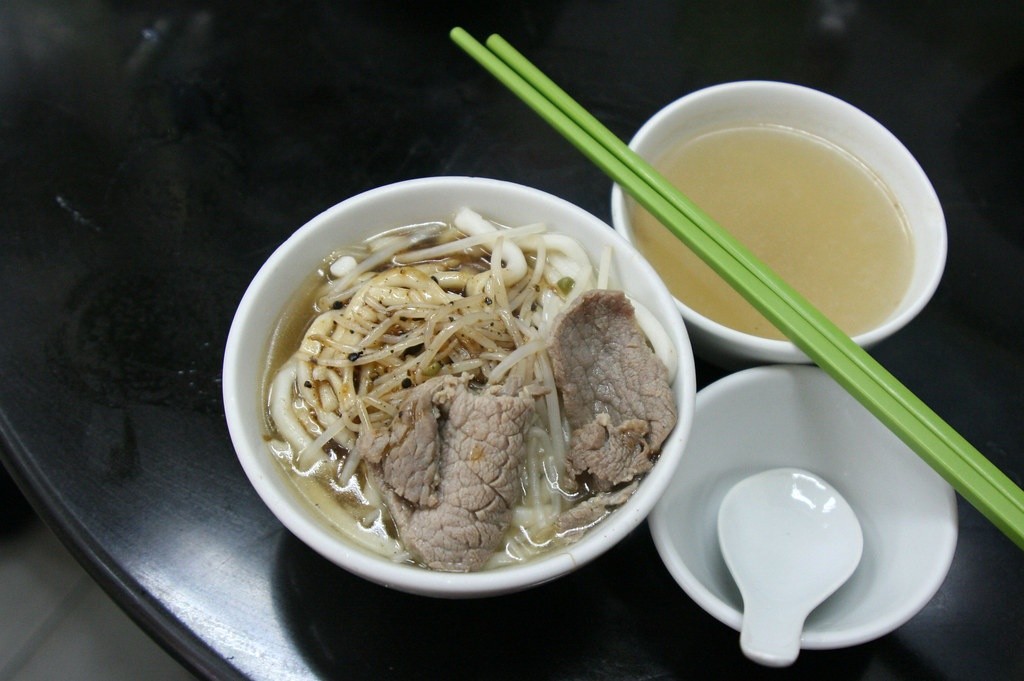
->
[611,81,948,365]
[646,365,958,649]
[222,176,697,599]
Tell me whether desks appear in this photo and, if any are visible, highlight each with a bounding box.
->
[0,0,1024,681]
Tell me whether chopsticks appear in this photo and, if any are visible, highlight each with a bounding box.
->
[451,26,1024,550]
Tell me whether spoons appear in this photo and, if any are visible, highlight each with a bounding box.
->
[716,468,863,666]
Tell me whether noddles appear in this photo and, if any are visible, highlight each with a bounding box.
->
[271,210,677,568]
